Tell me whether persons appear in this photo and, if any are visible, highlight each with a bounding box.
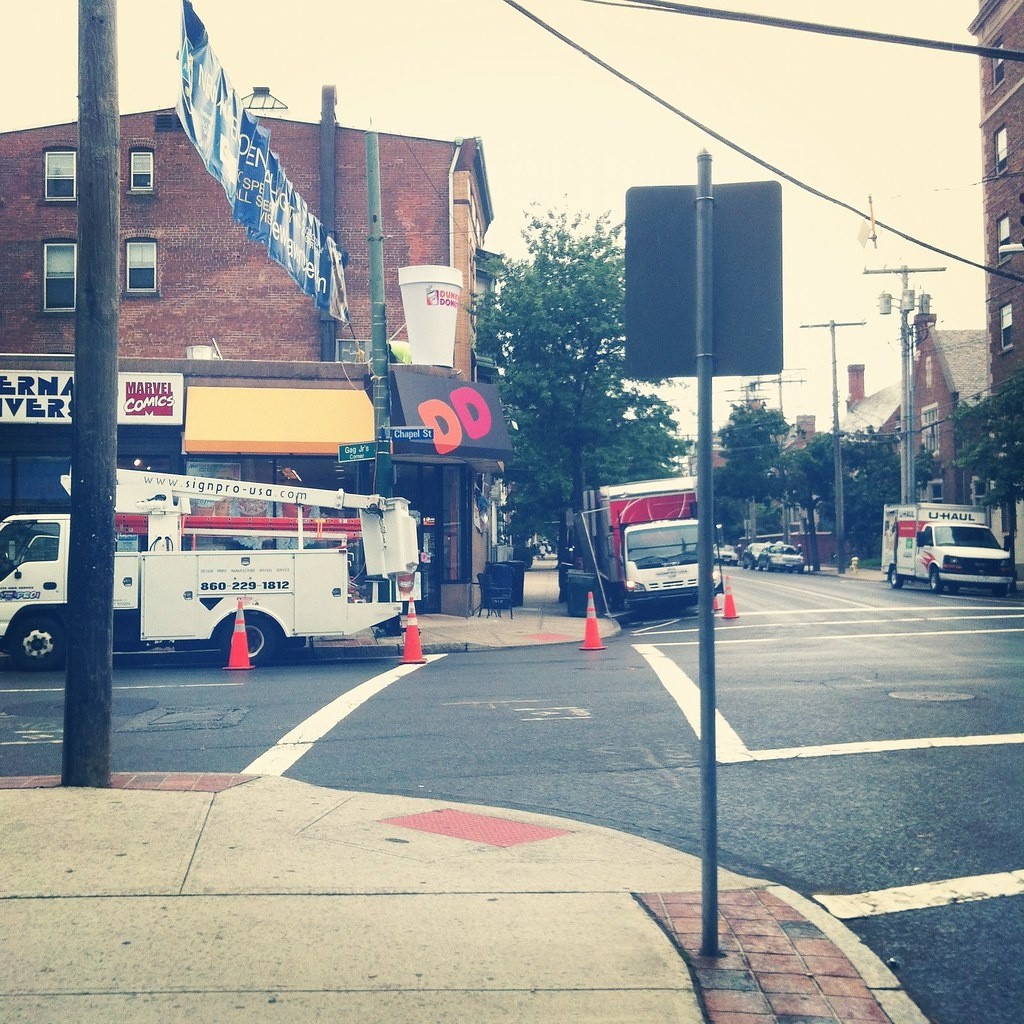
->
[539,544,551,559]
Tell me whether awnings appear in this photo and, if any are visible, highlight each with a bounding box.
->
[390,370,513,461]
[185,387,375,454]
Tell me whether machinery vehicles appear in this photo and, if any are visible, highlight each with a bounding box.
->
[0,466,423,677]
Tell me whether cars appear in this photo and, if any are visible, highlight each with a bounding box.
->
[713,544,739,566]
[742,542,774,569]
[757,541,806,574]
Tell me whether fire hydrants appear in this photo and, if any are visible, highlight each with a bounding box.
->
[850,557,859,575]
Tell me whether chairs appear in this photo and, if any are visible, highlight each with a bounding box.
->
[474,573,514,619]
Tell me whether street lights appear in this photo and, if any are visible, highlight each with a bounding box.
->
[878,289,934,502]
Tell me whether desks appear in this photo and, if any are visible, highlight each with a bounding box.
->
[472,582,499,616]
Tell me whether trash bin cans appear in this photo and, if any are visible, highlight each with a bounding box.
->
[484,561,525,607]
[566,572,601,617]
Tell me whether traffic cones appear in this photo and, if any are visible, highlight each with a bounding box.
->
[578,591,609,651]
[398,597,427,664]
[222,597,256,670]
[721,575,739,619]
[713,596,723,611]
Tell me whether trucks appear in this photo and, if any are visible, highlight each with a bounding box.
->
[581,473,723,611]
[882,502,1016,596]
